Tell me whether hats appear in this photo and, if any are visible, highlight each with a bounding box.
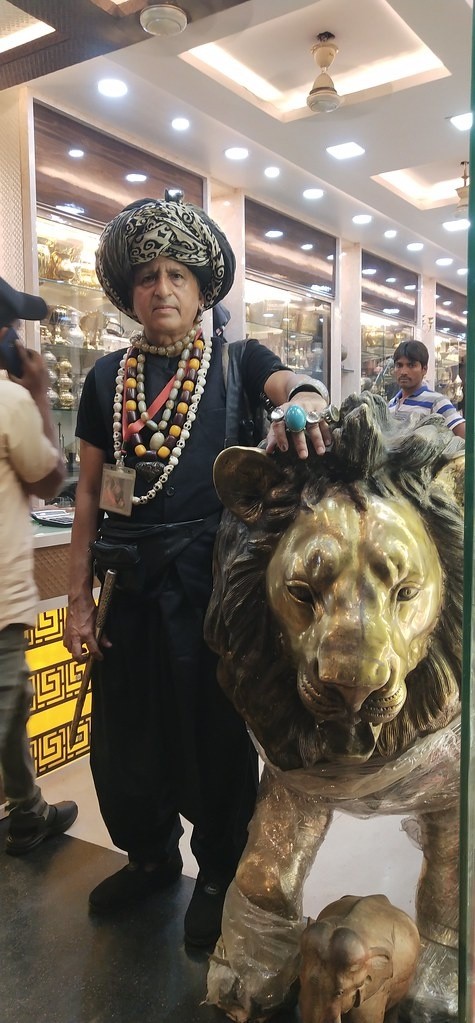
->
[0,278,47,326]
[95,188,235,326]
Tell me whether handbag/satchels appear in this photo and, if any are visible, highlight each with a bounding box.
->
[88,512,219,596]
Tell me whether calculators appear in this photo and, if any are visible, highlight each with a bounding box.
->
[30,509,75,528]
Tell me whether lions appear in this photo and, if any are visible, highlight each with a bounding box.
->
[202,388,465,1023]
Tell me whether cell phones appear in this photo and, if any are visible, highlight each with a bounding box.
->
[0,327,27,378]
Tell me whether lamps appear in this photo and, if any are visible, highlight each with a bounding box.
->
[455,161,469,210]
[307,33,339,113]
[140,3,187,37]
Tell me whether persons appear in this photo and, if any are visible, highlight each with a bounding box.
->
[386,340,466,441]
[0,276,78,860]
[62,197,332,962]
[104,476,126,508]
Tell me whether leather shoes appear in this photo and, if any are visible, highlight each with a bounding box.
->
[6,800,77,854]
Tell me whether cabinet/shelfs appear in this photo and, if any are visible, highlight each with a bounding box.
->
[363,342,464,408]
[244,319,323,387]
[38,276,140,491]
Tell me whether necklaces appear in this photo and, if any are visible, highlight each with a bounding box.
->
[112,321,213,506]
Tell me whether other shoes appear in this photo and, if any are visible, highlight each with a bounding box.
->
[184,871,232,945]
[88,848,182,914]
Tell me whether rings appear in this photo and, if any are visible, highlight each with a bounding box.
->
[270,403,341,434]
